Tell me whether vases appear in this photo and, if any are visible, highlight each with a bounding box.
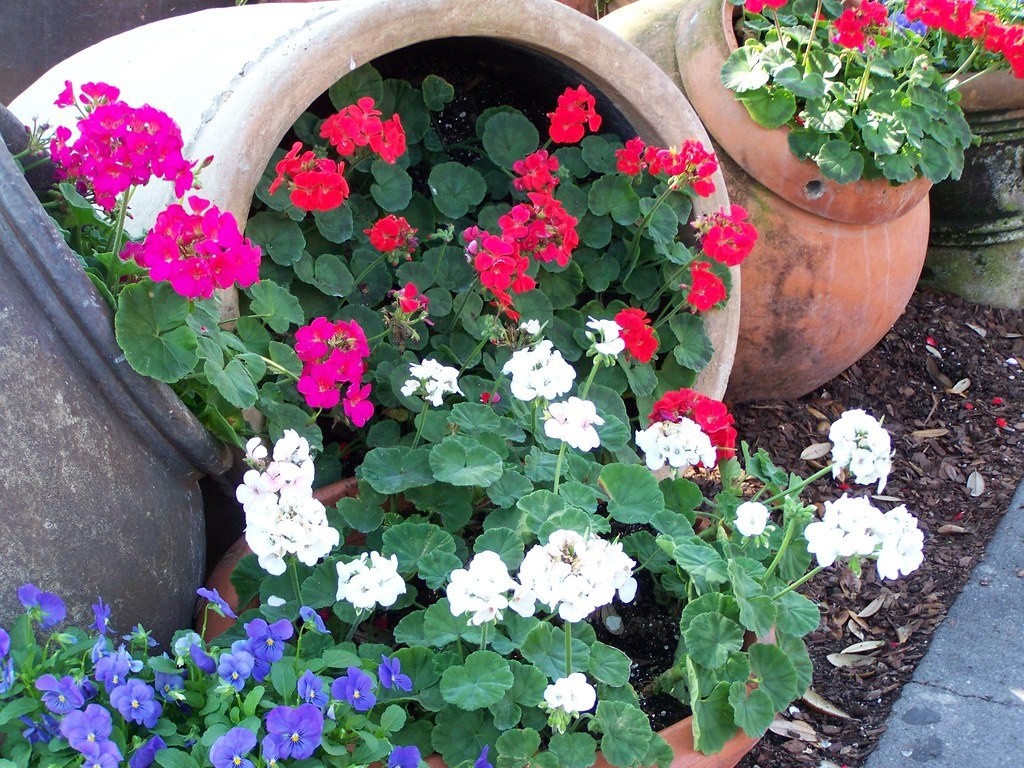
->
[0,0,740,431]
[0,99,240,672]
[595,0,935,413]
[930,65,1024,312]
[200,469,785,768]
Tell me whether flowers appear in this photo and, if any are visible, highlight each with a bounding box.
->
[0,0,1024,768]
